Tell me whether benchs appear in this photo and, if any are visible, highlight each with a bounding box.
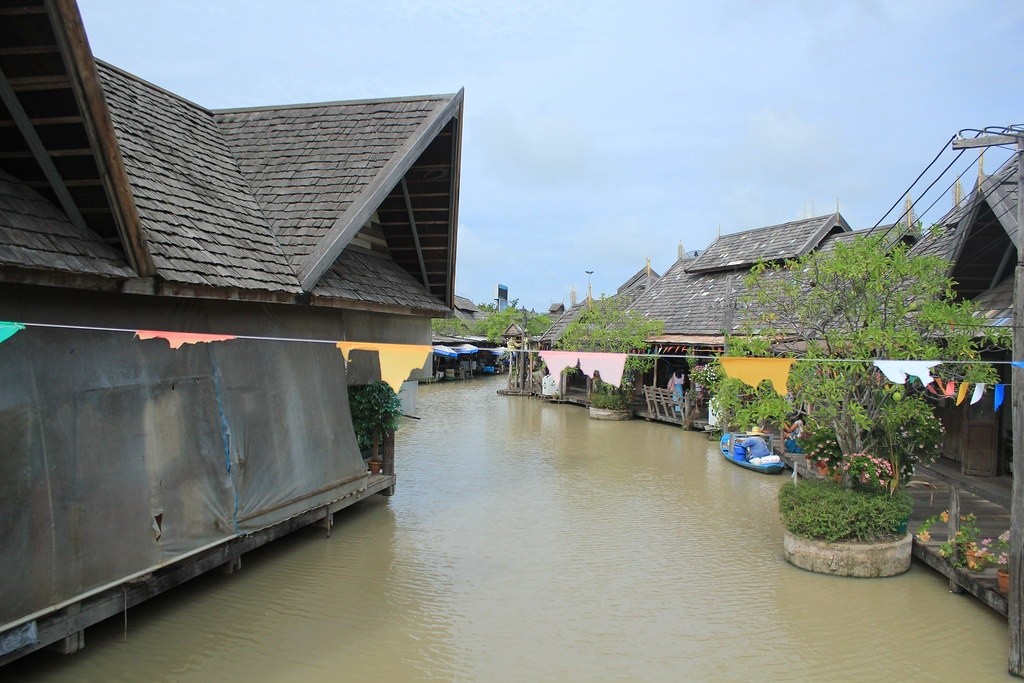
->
[643,384,698,423]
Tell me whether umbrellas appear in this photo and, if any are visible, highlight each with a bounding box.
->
[432,344,457,370]
[491,346,517,357]
[451,344,479,369]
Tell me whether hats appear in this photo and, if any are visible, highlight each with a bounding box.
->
[747,427,765,435]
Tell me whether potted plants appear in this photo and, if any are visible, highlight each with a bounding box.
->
[354,381,405,474]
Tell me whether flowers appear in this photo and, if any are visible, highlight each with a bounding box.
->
[795,415,894,487]
[913,509,1010,573]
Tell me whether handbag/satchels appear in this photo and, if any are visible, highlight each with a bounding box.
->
[667,376,674,391]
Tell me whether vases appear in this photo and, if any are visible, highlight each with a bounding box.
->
[836,475,843,484]
[861,470,871,483]
[998,568,1010,594]
[965,550,986,571]
[962,544,977,548]
[818,467,828,475]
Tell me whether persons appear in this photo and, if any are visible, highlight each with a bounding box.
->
[432,352,516,375]
[669,364,685,413]
[782,410,808,455]
[741,427,770,459]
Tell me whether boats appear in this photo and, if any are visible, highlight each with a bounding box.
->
[720,434,786,475]
[482,366,503,375]
[445,375,463,380]
